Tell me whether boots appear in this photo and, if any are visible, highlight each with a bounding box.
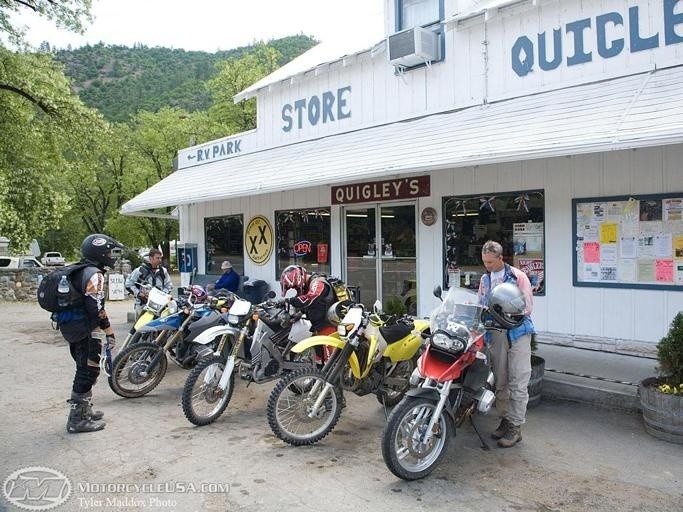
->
[66,390,105,433]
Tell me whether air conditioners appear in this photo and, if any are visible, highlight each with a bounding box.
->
[387,27,438,66]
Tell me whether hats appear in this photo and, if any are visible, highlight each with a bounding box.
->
[220,261,232,270]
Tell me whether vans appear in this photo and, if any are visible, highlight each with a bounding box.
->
[1,256,46,270]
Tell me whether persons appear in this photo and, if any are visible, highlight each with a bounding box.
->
[473,240,534,447]
[207,262,240,307]
[52,233,123,432]
[273,264,346,411]
[125,247,175,321]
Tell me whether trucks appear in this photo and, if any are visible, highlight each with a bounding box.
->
[42,252,66,266]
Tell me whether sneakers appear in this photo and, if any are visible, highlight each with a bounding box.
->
[497,423,521,448]
[491,420,508,439]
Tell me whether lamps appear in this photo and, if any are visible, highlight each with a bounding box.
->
[304,210,329,216]
[380,209,396,218]
[450,209,479,217]
[345,209,368,218]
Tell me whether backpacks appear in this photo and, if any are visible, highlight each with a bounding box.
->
[306,273,352,301]
[37,262,97,312]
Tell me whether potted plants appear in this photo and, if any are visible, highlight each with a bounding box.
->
[527,333,545,408]
[640,312,682,444]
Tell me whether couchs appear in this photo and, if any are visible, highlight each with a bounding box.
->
[190,275,248,297]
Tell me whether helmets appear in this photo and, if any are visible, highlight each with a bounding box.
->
[279,265,306,290]
[193,285,207,303]
[81,234,124,269]
[488,282,532,329]
[327,300,356,327]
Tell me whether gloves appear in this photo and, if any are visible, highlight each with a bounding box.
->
[137,292,147,306]
[273,297,284,309]
[105,334,114,350]
